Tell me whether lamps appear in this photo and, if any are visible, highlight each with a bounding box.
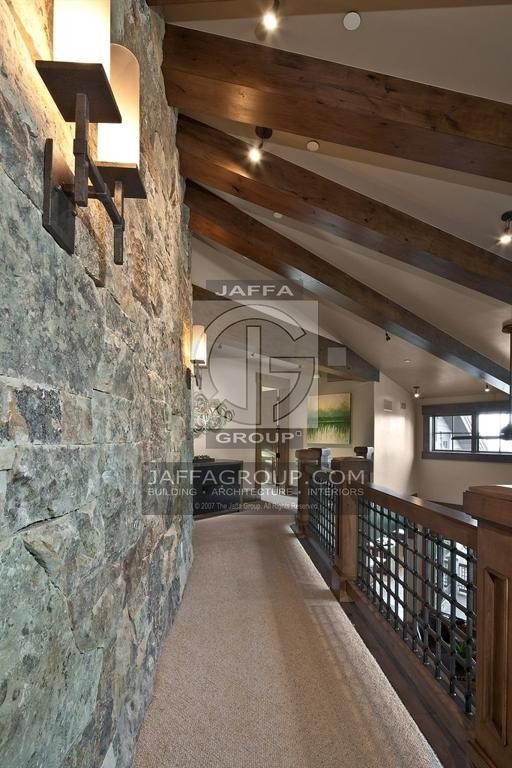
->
[31,1,149,267]
[185,325,208,390]
